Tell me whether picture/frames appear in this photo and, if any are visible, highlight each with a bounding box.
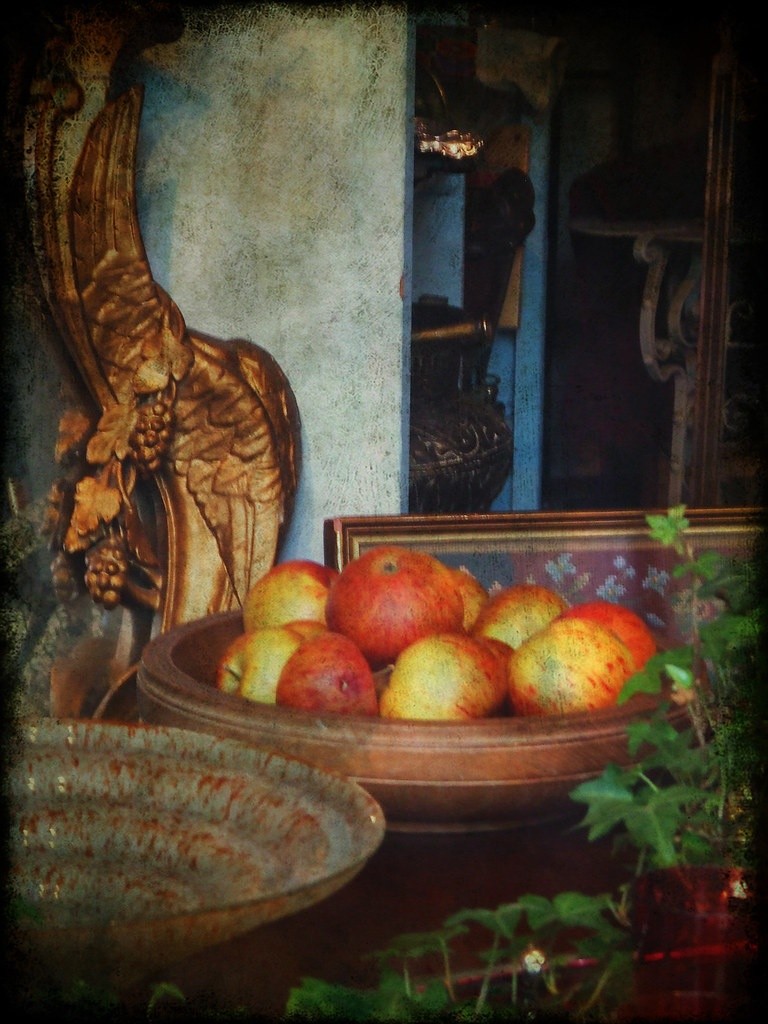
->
[326,504,764,688]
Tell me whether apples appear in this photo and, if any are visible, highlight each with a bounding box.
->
[215,545,656,719]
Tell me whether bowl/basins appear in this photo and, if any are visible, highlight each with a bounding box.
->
[0,718,386,992]
[134,606,705,835]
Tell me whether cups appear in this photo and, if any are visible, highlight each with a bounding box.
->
[630,864,768,1023]
